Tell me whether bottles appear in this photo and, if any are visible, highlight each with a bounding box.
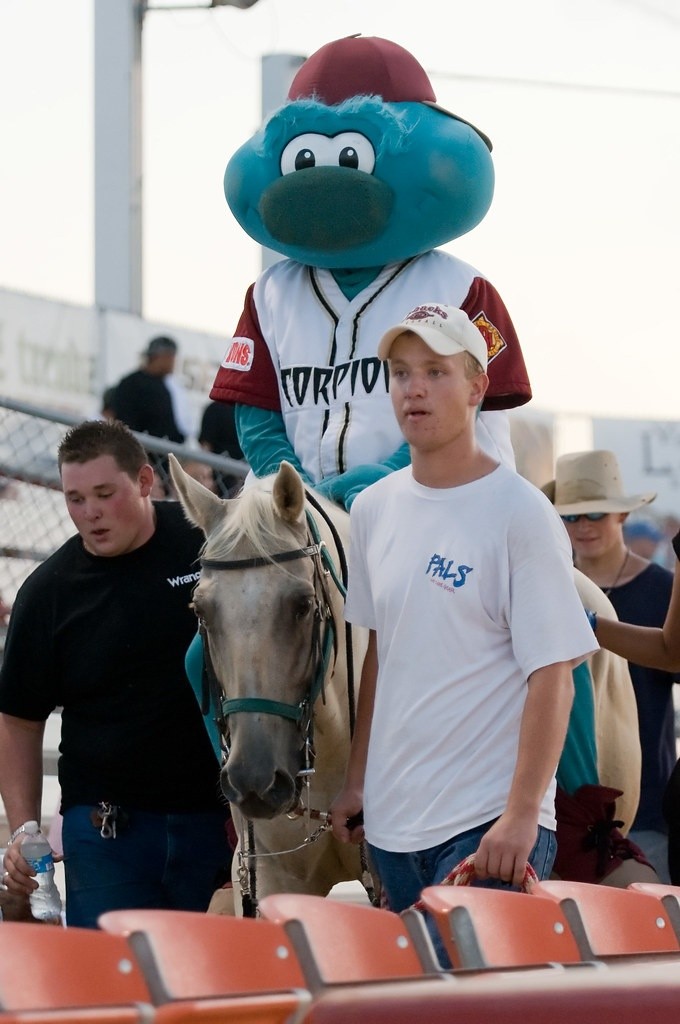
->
[20,821,63,919]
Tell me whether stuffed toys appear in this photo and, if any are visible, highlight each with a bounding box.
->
[184,36,652,883]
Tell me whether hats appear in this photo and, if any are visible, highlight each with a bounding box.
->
[377,303,487,374]
[540,450,657,516]
[623,521,664,543]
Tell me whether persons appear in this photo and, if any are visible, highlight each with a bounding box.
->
[539,450,680,831]
[102,336,184,496]
[196,400,246,500]
[585,528,680,673]
[330,304,602,969]
[0,420,234,929]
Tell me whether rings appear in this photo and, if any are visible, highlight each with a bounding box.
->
[4,872,9,876]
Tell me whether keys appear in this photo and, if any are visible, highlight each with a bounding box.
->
[97,806,117,839]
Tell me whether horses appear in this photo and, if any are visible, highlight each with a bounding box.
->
[164,452,642,916]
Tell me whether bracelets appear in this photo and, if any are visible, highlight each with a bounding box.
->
[584,608,597,632]
[11,825,41,844]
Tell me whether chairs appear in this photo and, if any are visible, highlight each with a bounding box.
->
[0,875,680,1024]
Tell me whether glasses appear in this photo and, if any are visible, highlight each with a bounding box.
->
[560,512,606,523]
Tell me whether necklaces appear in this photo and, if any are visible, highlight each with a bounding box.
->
[605,548,630,597]
